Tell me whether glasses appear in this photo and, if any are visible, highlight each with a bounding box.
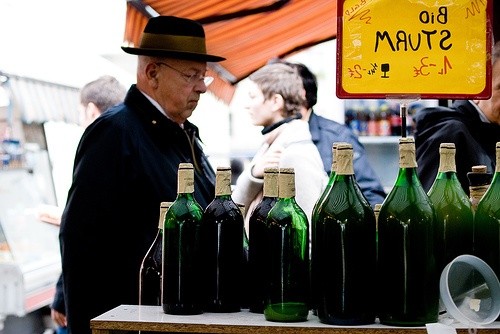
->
[155,62,210,82]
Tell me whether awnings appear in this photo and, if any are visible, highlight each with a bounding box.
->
[124,0,337,105]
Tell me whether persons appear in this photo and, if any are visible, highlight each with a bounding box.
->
[49,16,227,334]
[50,76,128,334]
[287,63,388,209]
[413,41,500,193]
[231,63,330,260]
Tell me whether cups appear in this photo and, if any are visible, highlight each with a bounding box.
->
[438,254,500,326]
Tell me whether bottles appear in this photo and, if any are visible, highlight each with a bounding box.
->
[379,137,436,326]
[162,162,204,316]
[345,105,400,137]
[310,143,344,316]
[265,167,310,321]
[316,143,377,325]
[248,169,278,313]
[473,142,500,322]
[204,167,242,313]
[374,204,381,264]
[232,202,247,311]
[427,142,473,323]
[467,165,494,213]
[138,201,174,334]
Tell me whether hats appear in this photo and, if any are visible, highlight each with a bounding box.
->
[120,15,226,62]
